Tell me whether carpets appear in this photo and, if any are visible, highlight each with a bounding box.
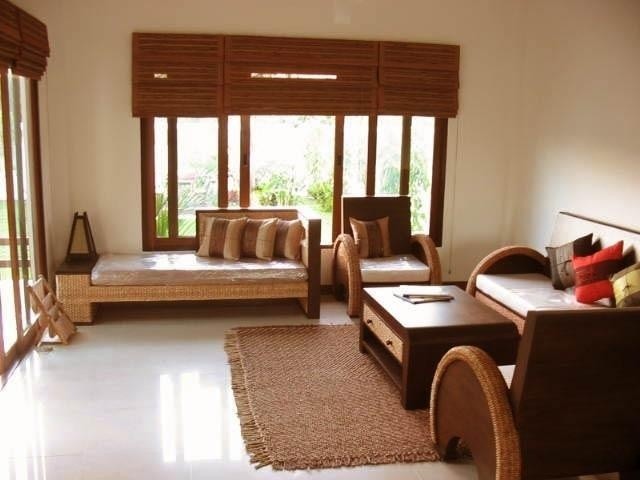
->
[223,324,474,472]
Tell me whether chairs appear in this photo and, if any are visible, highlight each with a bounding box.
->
[331,196,442,317]
[428,308,639,480]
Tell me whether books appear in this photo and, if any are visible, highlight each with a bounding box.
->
[399,284,455,297]
[393,291,452,305]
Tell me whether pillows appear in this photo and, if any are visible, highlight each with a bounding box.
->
[195,216,304,261]
[544,233,640,309]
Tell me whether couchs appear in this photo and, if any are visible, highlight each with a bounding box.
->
[466,211,640,335]
[53,209,322,325]
